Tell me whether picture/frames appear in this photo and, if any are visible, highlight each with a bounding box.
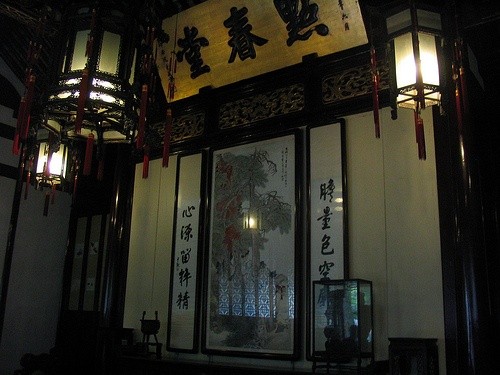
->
[168,147,205,355]
[202,123,304,363]
[303,120,348,362]
